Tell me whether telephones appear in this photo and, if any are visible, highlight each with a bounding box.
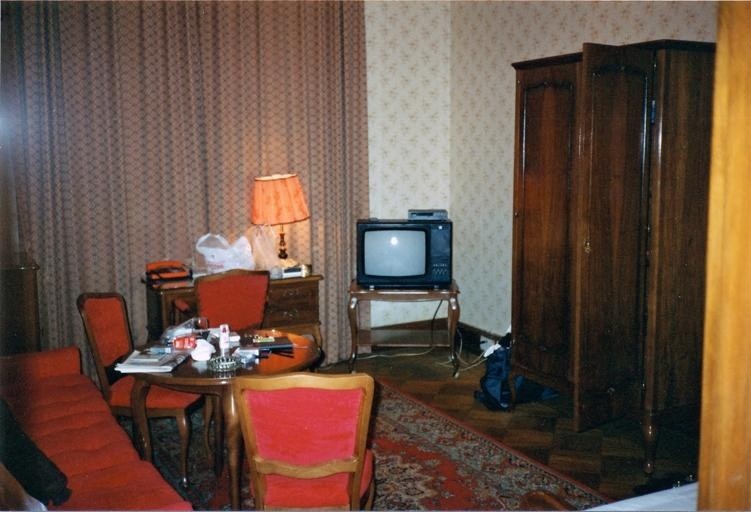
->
[146,261,192,280]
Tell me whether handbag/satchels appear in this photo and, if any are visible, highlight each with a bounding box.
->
[477,333,557,413]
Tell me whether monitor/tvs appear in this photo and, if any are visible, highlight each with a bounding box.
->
[356,219,453,290]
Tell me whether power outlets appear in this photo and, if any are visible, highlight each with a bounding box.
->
[479,335,495,351]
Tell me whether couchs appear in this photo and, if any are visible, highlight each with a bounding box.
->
[0,346,196,512]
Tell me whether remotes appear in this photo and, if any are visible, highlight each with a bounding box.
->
[369,217,378,221]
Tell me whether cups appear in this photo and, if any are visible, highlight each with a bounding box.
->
[192,317,208,338]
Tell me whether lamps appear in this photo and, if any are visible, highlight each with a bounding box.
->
[251,173,311,260]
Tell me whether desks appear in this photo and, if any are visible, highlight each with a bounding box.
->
[0,251,43,352]
[346,278,461,367]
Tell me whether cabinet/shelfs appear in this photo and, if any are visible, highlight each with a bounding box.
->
[507,39,716,474]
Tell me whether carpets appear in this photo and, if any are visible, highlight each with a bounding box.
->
[117,365,616,512]
